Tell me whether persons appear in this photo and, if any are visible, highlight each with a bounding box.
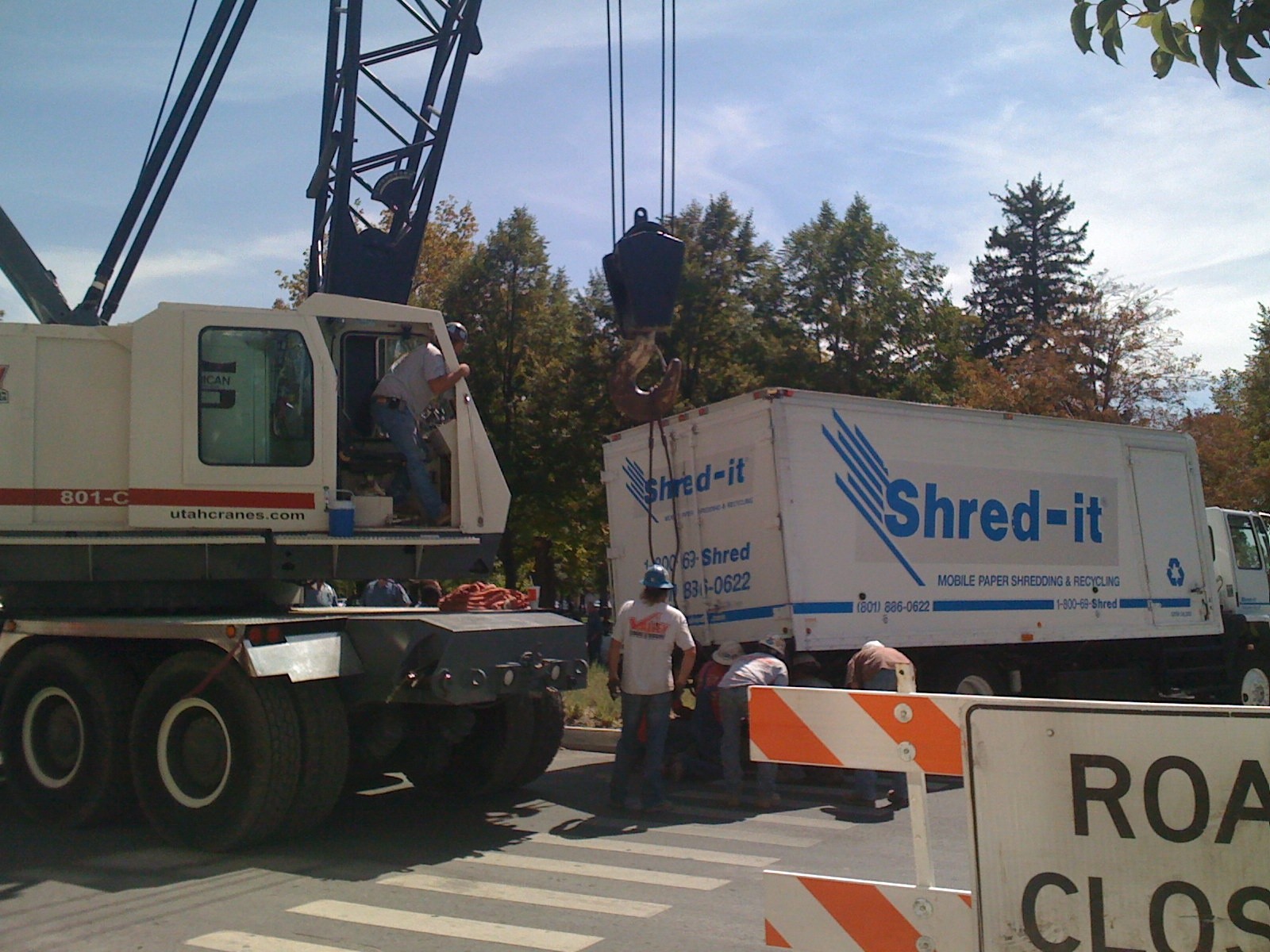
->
[622,633,834,808]
[841,640,916,809]
[608,564,696,814]
[370,321,470,527]
[300,577,446,607]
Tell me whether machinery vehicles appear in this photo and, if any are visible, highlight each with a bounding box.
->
[0,0,687,858]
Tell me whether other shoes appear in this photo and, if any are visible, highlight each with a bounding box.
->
[393,504,416,513]
[435,505,452,526]
[888,790,907,808]
[608,800,623,810]
[641,800,673,813]
[758,791,781,806]
[841,788,876,808]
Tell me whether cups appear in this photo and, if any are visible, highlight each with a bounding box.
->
[526,586,540,608]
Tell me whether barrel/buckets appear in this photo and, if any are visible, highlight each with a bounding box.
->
[326,490,356,537]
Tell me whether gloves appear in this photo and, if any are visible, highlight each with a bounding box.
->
[607,677,621,701]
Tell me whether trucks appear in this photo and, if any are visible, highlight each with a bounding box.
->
[599,386,1270,789]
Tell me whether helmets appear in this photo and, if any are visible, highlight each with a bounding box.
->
[712,640,746,667]
[861,641,884,651]
[639,564,674,589]
[758,634,786,655]
[446,321,468,344]
